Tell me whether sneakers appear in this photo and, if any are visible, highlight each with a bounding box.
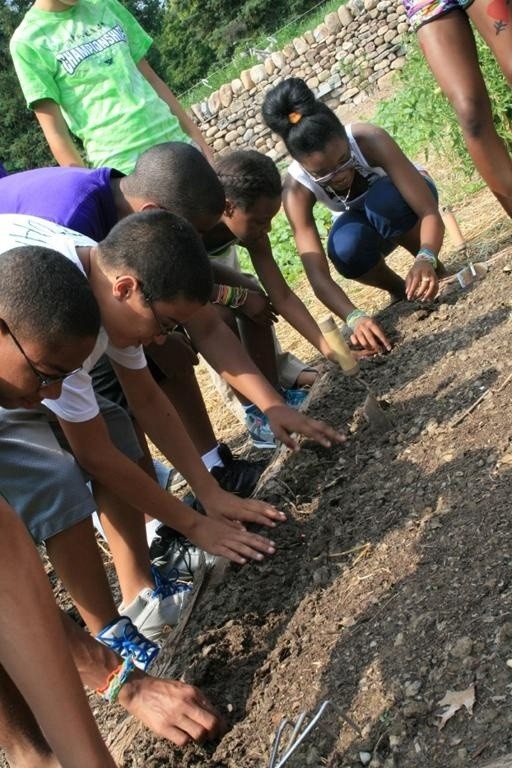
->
[290,361,324,389]
[149,524,218,574]
[116,578,193,640]
[245,389,308,450]
[191,442,269,536]
[91,614,161,673]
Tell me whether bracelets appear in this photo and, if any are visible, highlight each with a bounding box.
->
[95,653,139,706]
[409,245,439,272]
[341,306,369,330]
[210,282,250,310]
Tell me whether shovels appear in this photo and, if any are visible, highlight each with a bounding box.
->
[317,315,395,435]
[440,206,488,288]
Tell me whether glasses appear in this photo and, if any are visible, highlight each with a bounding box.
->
[116,276,180,340]
[2,317,83,391]
[300,142,356,184]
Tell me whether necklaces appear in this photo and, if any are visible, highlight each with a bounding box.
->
[324,184,352,212]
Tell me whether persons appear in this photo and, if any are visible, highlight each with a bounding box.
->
[170,148,380,452]
[0,490,116,766]
[398,0,512,216]
[0,205,291,642]
[6,1,248,423]
[260,76,447,359]
[0,242,232,755]
[0,139,348,568]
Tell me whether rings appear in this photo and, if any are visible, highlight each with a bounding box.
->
[419,275,431,282]
